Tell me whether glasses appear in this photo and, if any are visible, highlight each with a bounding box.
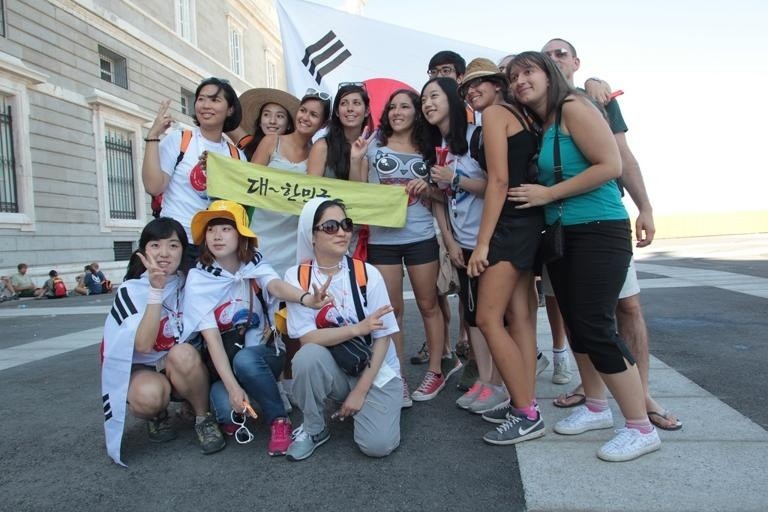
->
[338,81,367,92]
[543,48,574,59]
[426,67,458,78]
[312,217,354,235]
[305,87,333,114]
[230,407,255,445]
[460,76,493,100]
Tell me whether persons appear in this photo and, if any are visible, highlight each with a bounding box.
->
[102,38,684,462]
[1,263,106,300]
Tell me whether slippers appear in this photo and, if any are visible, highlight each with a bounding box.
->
[553,392,587,408]
[646,408,683,431]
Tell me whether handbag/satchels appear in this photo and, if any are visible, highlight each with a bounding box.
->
[323,323,374,377]
[201,326,247,376]
[435,231,462,296]
[537,216,567,265]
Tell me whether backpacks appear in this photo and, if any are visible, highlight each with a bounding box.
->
[102,280,113,293]
[53,277,66,298]
[150,194,163,219]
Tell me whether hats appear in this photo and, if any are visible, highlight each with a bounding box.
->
[237,87,303,136]
[189,199,259,249]
[456,57,510,100]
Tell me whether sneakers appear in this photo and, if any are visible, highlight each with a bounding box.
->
[595,426,662,462]
[455,339,471,368]
[267,415,295,457]
[467,382,511,414]
[410,341,430,364]
[455,379,486,410]
[481,400,542,424]
[535,351,550,376]
[552,404,614,436]
[440,351,464,381]
[285,423,333,462]
[193,412,227,455]
[482,410,547,446]
[401,375,413,408]
[175,400,196,424]
[146,409,177,443]
[220,421,242,436]
[410,370,447,402]
[552,351,572,385]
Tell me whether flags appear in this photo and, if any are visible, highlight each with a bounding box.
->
[276,1,508,144]
